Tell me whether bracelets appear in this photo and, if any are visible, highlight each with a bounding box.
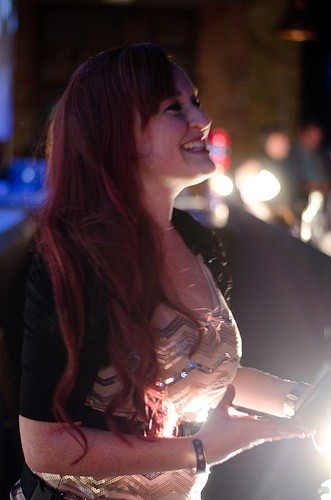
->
[190,434,208,478]
[283,377,309,419]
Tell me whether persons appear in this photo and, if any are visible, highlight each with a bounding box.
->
[6,42,311,500]
